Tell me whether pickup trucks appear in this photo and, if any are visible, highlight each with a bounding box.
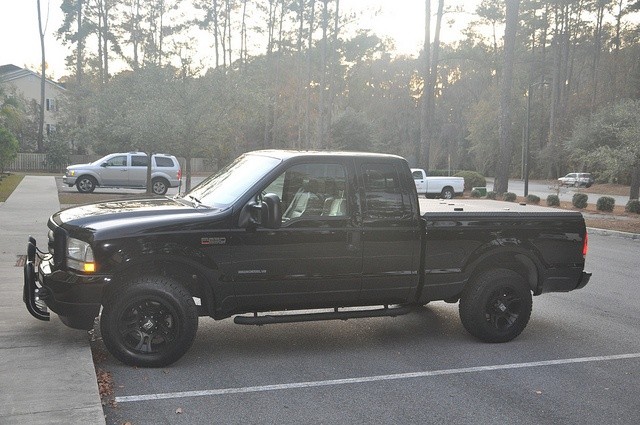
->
[21,150,592,369]
[412,167,465,198]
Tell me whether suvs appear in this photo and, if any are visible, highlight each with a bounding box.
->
[554,169,593,189]
[62,147,183,195]
